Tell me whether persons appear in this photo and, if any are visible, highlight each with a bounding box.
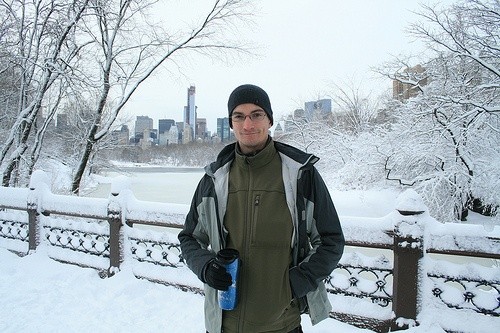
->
[177,85,346,333]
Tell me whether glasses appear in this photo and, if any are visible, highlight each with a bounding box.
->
[229,112,268,121]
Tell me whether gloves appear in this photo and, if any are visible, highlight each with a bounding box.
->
[205,260,232,291]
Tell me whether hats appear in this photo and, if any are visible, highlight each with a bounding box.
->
[227,84,273,129]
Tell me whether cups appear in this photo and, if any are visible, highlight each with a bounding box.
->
[214,248,240,310]
